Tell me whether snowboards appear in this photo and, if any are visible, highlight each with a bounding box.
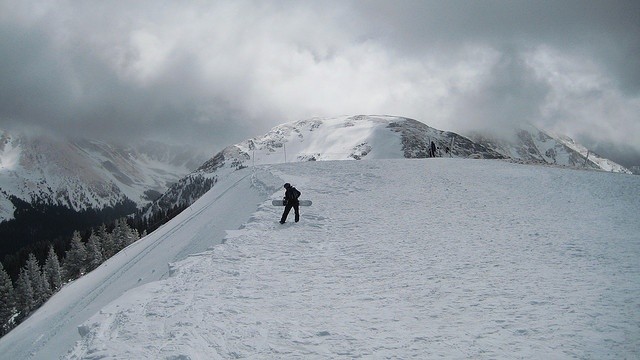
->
[272,199,312,206]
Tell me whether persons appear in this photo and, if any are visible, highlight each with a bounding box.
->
[431,141,435,157]
[280,183,301,224]
